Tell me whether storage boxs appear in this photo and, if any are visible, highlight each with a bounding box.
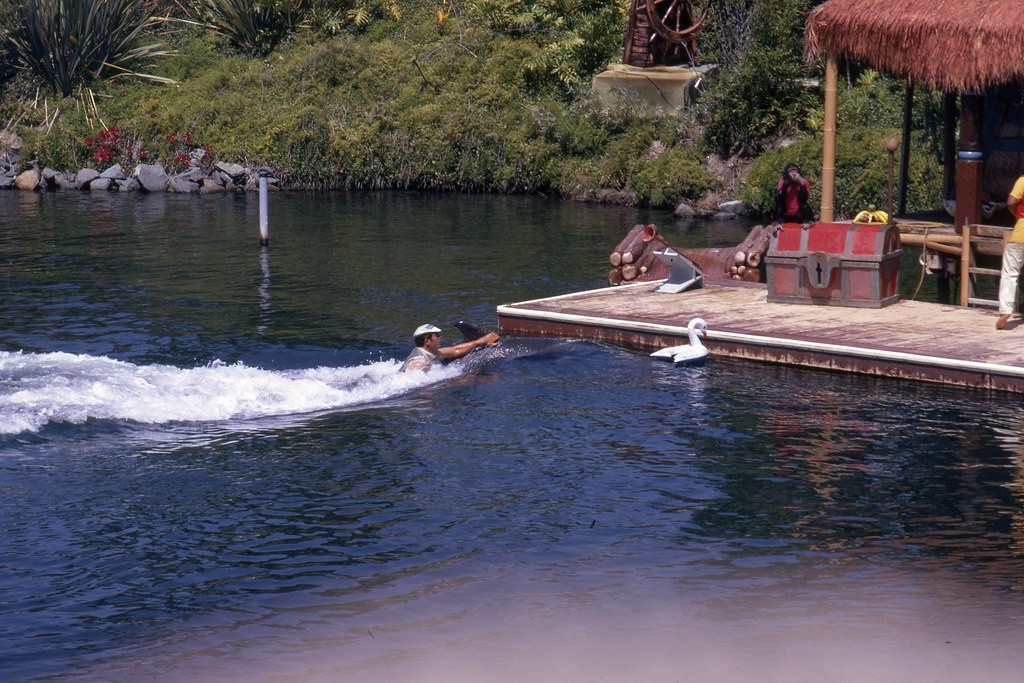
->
[764,222,904,309]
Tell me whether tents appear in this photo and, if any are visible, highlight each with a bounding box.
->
[804,0,1024,222]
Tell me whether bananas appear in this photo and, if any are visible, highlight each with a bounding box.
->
[854,210,889,223]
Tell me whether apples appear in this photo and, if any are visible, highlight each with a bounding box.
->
[860,215,869,223]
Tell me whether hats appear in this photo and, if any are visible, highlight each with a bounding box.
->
[414,324,441,335]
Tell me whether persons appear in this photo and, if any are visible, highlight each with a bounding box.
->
[996,175,1024,329]
[400,323,500,371]
[775,163,815,224]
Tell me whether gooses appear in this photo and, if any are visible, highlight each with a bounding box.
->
[650,317,708,364]
